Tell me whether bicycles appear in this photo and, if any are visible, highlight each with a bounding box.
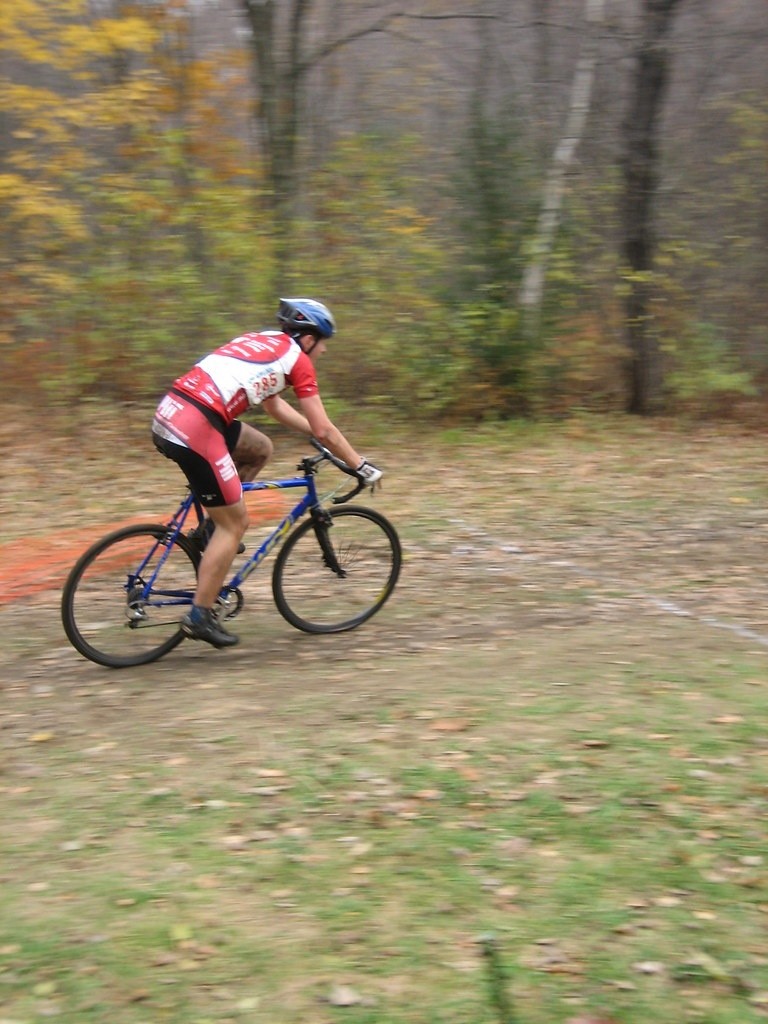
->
[61,436,401,668]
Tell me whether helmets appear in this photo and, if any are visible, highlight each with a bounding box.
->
[274,298,337,338]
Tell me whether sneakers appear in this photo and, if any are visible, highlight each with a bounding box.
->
[181,616,238,648]
[189,519,246,555]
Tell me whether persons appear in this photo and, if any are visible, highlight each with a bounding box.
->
[152,297,383,648]
